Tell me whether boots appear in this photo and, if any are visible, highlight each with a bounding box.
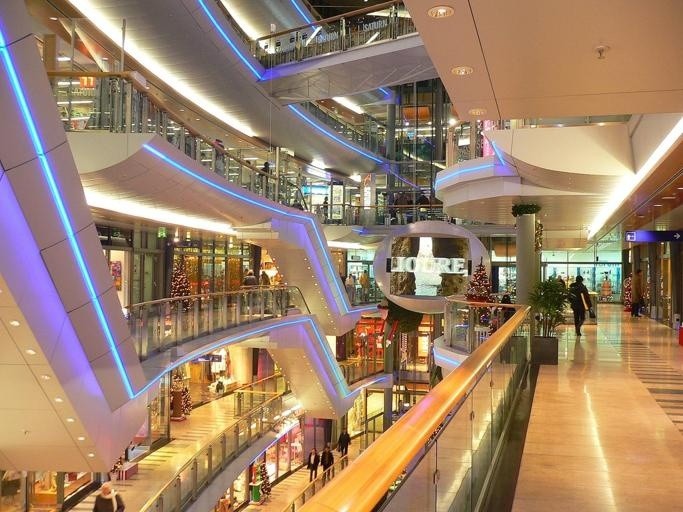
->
[575,321,584,336]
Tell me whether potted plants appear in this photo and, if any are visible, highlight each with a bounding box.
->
[527,277,576,365]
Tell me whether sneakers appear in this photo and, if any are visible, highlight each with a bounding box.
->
[631,311,642,318]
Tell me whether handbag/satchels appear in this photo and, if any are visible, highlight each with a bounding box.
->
[639,294,649,307]
[580,283,590,312]
[589,308,596,318]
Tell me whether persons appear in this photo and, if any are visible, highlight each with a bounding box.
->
[358,269,371,302]
[155,124,445,226]
[305,447,318,482]
[567,275,593,335]
[554,274,565,290]
[237,264,357,316]
[337,427,350,458]
[92,482,124,512]
[491,294,516,364]
[319,446,334,481]
[630,269,646,319]
